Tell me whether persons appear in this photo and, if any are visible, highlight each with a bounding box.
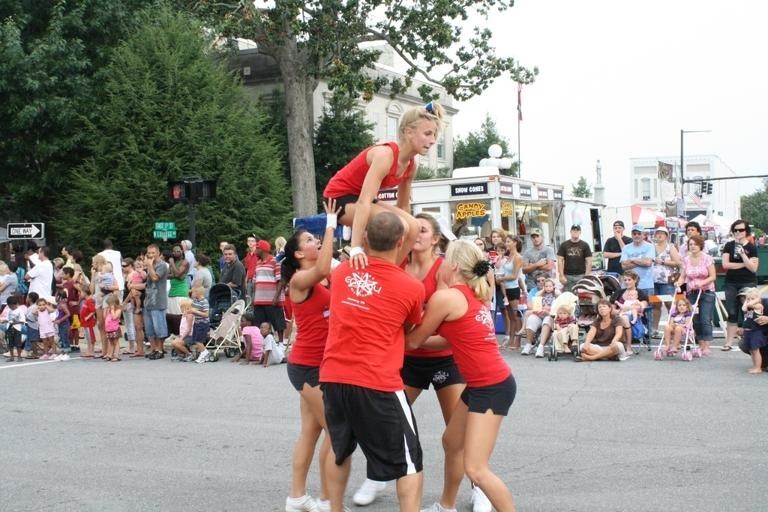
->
[474,219,768,374]
[318,210,427,512]
[595,157,602,184]
[352,215,495,511]
[278,196,351,512]
[322,100,445,270]
[0,232,298,364]
[404,238,519,511]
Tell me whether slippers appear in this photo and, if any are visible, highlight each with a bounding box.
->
[721,344,732,351]
[94,351,143,361]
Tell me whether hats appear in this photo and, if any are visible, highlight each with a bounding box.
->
[613,220,625,227]
[255,239,271,253]
[630,224,644,232]
[529,227,542,236]
[654,226,669,237]
[571,224,581,231]
[734,287,750,305]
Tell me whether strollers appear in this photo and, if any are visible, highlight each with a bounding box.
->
[654,283,701,361]
[193,283,251,361]
[573,271,621,332]
[547,291,580,361]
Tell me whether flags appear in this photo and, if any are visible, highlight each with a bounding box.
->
[517,82,524,124]
[658,161,674,181]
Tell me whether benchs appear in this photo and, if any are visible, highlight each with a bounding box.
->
[517,303,654,351]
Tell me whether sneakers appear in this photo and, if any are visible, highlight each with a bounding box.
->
[145,349,211,364]
[521,343,535,355]
[352,478,387,505]
[515,330,526,336]
[470,484,493,512]
[284,493,313,512]
[535,343,545,358]
[312,497,350,512]
[3,345,80,362]
[421,501,457,512]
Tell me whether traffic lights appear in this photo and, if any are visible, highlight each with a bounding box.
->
[701,181,707,194]
[168,177,215,202]
[707,182,713,194]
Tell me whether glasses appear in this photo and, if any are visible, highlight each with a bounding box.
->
[122,264,129,267]
[733,228,744,233]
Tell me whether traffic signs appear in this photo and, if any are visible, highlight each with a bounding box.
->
[7,221,45,240]
[152,221,177,239]
